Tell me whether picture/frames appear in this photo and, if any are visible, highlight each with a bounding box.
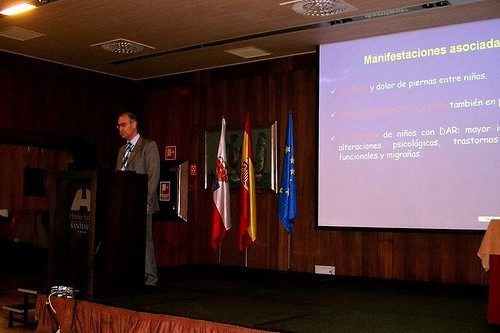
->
[199,120,279,195]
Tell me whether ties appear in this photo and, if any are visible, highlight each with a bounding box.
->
[121,143,134,170]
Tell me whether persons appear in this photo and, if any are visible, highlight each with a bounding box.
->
[115,113,161,286]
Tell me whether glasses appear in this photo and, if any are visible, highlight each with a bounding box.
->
[116,122,131,128]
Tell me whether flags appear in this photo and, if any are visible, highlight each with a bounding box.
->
[239,111,257,250]
[278,109,298,234]
[209,115,232,249]
